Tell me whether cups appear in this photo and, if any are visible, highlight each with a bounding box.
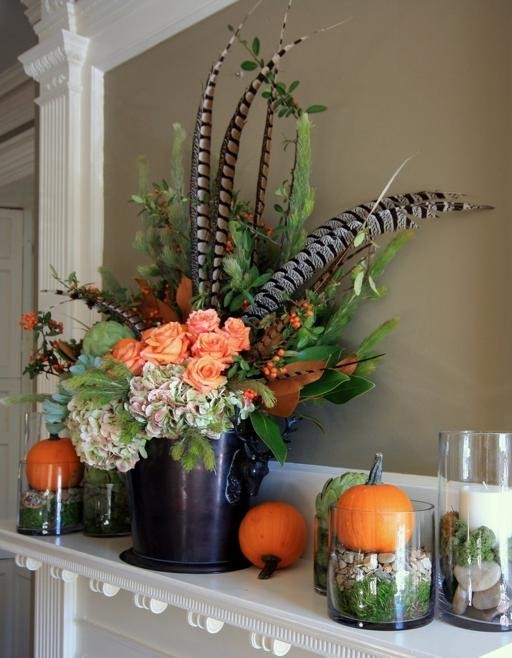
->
[314,430,512,631]
[16,410,129,538]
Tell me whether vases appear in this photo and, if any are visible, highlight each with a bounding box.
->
[119,417,295,574]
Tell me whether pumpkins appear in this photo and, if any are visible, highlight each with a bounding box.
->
[238,502,308,580]
[332,452,415,553]
[26,421,84,490]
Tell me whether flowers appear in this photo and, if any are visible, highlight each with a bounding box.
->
[0,0,495,474]
